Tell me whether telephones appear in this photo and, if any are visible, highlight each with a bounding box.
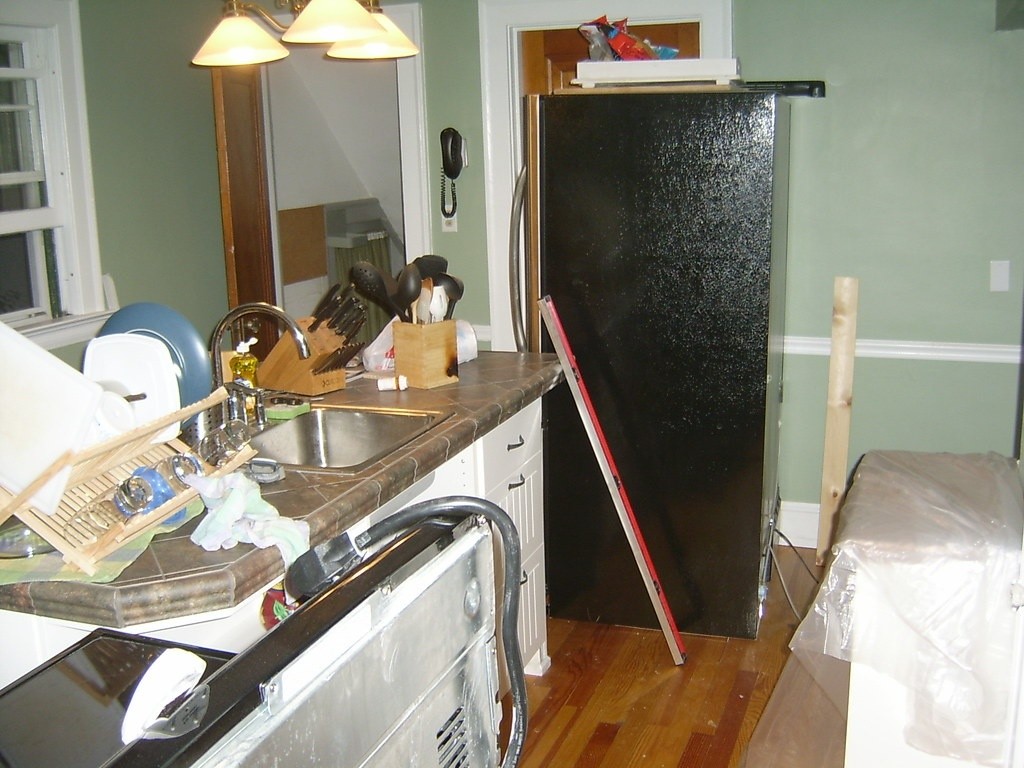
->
[440,127,463,179]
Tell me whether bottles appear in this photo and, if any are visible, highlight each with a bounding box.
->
[64,419,249,538]
[228,337,258,424]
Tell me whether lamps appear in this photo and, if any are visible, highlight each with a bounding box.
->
[190,0,419,68]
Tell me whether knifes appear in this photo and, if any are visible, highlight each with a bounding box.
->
[307,281,369,376]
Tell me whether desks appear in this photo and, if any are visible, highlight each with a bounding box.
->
[833,450,1024,768]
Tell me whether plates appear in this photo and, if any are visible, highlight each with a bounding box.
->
[99,302,213,430]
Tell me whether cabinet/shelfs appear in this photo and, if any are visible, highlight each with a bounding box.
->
[541,90,792,639]
[475,396,552,676]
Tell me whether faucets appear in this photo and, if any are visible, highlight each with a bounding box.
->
[208,302,311,391]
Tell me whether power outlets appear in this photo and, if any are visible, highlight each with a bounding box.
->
[441,204,457,232]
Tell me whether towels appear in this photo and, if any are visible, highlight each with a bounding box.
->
[184,470,311,606]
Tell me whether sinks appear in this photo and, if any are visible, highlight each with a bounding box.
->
[236,403,456,476]
